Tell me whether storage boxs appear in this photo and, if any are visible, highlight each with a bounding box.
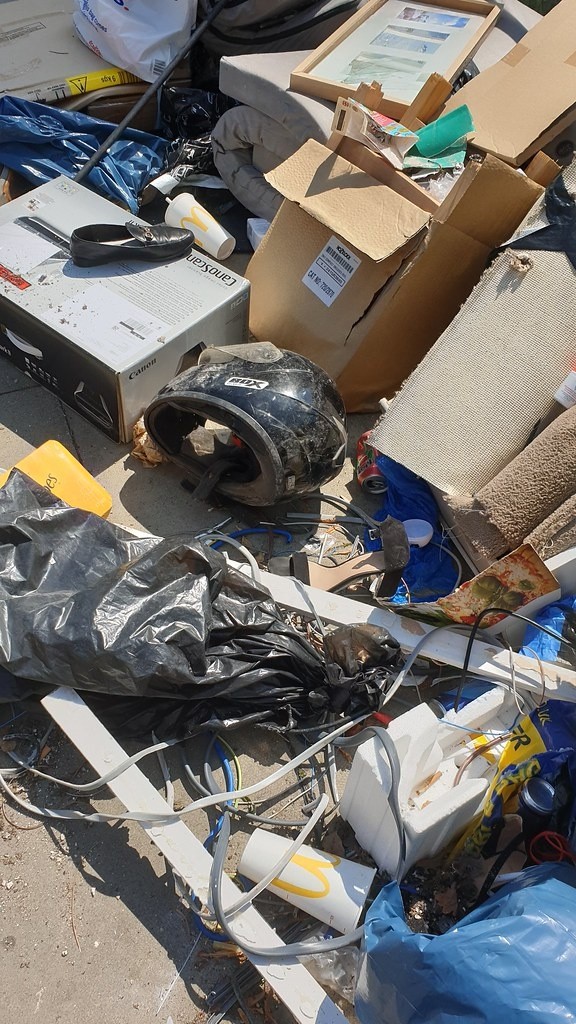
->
[0,174,252,444]
[245,113,549,413]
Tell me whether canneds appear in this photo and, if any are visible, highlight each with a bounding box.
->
[501,778,556,845]
[430,680,494,720]
[356,429,388,494]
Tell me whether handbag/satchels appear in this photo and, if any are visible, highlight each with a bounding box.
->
[71,0,197,84]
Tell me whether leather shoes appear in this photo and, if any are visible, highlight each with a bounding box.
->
[70,223,196,266]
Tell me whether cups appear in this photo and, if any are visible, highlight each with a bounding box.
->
[164,193,236,260]
[554,370,576,409]
[238,828,378,935]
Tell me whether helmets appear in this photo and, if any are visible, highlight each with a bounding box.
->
[143,348,347,507]
